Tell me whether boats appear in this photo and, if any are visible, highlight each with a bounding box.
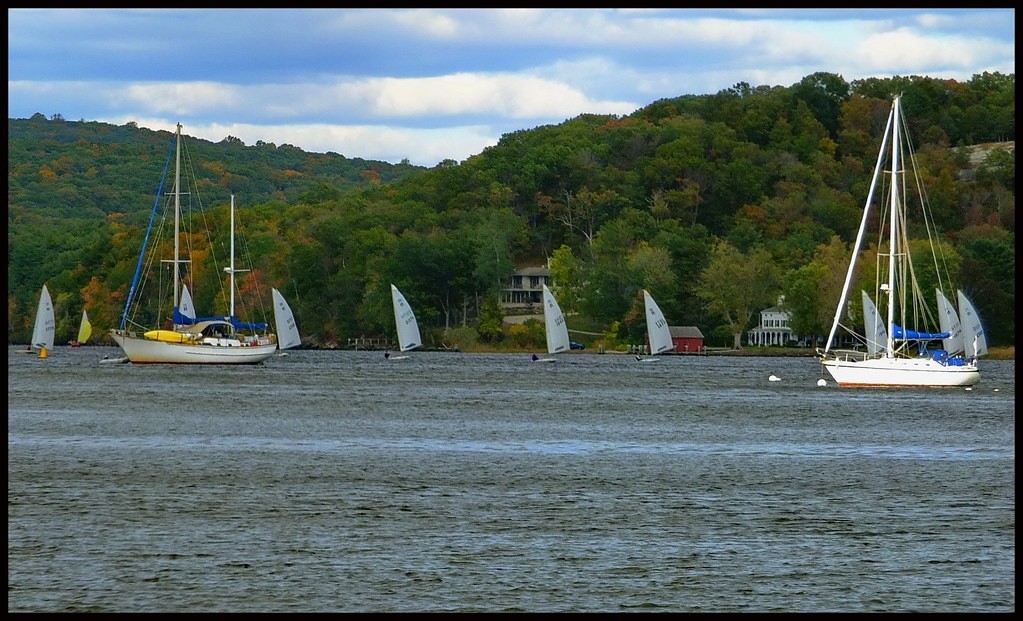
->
[97,356,129,365]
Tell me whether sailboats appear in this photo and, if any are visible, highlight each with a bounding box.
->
[815,289,888,361]
[634,287,674,362]
[14,285,55,356]
[531,283,570,362]
[387,283,424,360]
[823,97,985,387]
[271,286,302,355]
[113,124,278,365]
[68,310,93,347]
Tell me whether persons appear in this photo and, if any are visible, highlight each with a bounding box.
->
[254,332,259,341]
[636,353,643,361]
[532,354,538,361]
[384,351,389,359]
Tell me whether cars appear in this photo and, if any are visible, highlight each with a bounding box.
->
[569,341,585,351]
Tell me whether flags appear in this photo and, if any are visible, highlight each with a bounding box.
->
[973,335,977,356]
